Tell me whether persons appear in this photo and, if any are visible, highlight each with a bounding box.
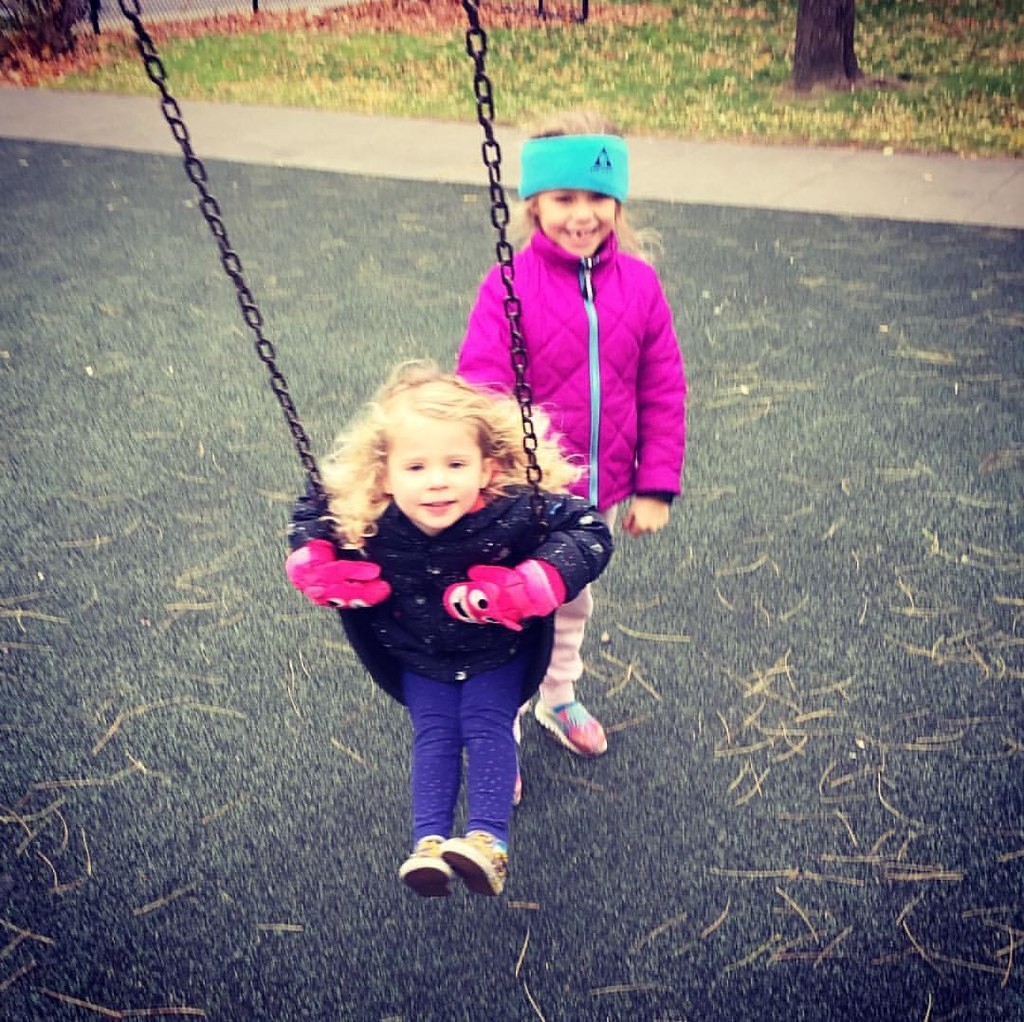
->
[284,360,615,901]
[454,112,686,756]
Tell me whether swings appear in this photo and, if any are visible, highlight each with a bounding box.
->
[116,0,557,711]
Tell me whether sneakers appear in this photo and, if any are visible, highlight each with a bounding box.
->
[535,695,607,756]
[398,835,454,898]
[511,759,521,805]
[441,829,508,895]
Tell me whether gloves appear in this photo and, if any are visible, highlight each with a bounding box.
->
[444,558,559,631]
[286,539,390,610]
